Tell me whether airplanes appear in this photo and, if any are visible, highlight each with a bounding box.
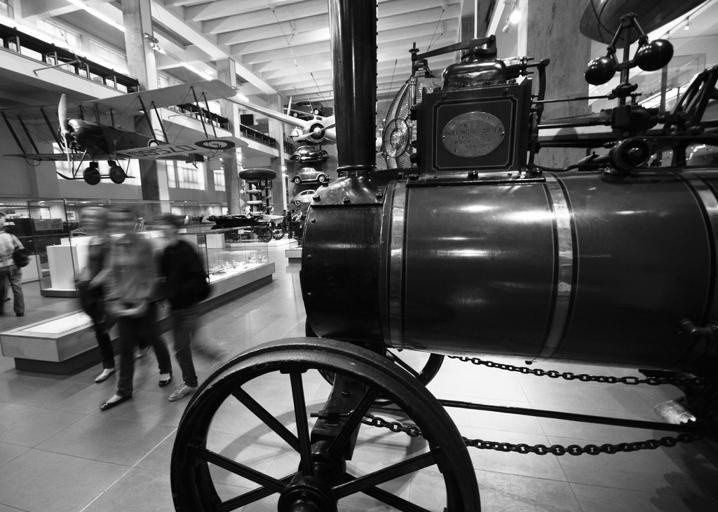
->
[222,86,387,156]
[0,74,253,189]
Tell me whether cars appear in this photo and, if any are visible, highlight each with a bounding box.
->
[286,99,332,213]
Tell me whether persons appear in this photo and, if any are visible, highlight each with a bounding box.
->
[154,212,221,401]
[101,209,172,412]
[0,220,25,317]
[0,212,11,302]
[75,206,149,384]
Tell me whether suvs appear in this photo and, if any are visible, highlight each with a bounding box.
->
[151,0,718,511]
[204,212,286,244]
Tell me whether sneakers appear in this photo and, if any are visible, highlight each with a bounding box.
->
[166,377,199,401]
[93,364,118,383]
[99,386,134,411]
[157,367,173,388]
